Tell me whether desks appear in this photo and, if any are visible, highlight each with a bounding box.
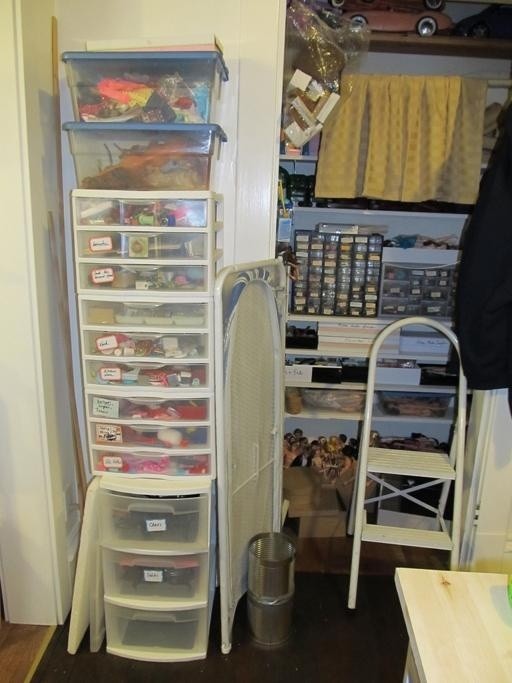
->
[393,567,512,683]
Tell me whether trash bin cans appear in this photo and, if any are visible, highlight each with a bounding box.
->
[247,532,297,650]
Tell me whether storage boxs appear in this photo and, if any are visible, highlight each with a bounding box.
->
[375,473,452,535]
[62,122,227,190]
[61,50,229,124]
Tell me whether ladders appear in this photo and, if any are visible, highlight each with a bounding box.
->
[342,317,467,616]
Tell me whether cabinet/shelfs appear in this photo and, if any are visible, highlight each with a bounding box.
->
[69,188,223,481]
[98,477,216,664]
[284,32,512,579]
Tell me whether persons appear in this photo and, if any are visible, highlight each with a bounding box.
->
[284,427,380,488]
